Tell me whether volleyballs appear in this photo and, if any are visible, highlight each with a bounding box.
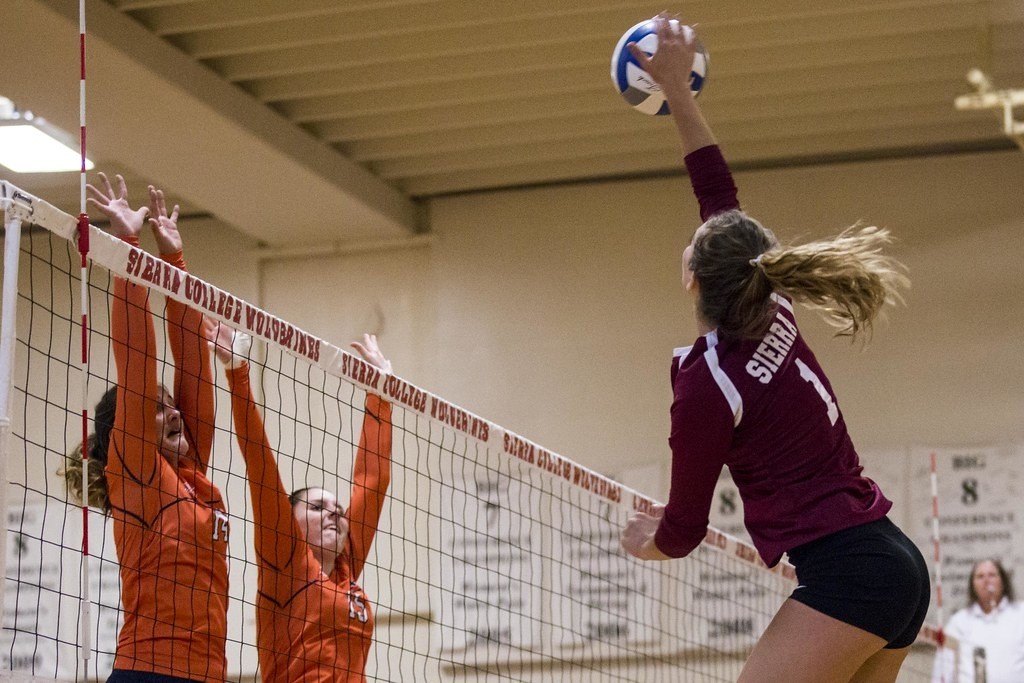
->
[608,15,712,119]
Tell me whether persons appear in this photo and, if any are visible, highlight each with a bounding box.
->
[203,316,393,683]
[58,171,230,683]
[931,557,1024,683]
[621,11,931,683]
